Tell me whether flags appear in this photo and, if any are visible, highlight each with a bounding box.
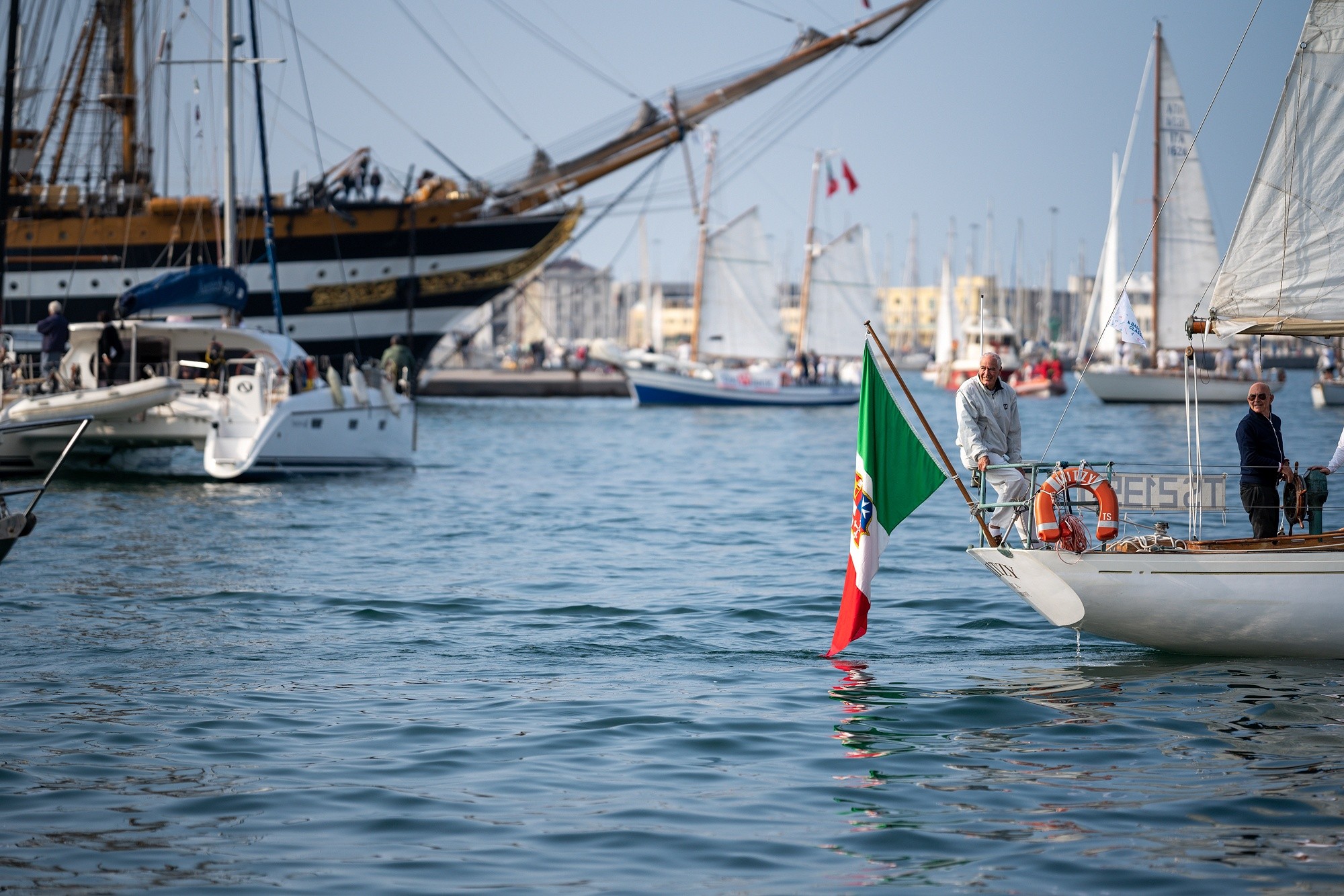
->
[820,156,839,196]
[840,158,858,193]
[823,336,948,666]
[1110,290,1147,348]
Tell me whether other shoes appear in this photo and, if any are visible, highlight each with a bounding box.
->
[982,523,1005,548]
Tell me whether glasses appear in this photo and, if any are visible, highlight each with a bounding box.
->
[1248,393,1271,400]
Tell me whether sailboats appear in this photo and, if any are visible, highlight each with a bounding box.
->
[0,1,945,575]
[964,1,1342,663]
[922,17,1343,407]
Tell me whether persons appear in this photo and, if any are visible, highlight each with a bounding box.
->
[97,311,124,386]
[1111,338,1137,369]
[380,334,419,394]
[956,349,1063,549]
[786,349,843,388]
[1307,423,1344,475]
[1156,346,1180,371]
[37,301,69,396]
[1320,342,1336,382]
[1235,382,1294,540]
[1213,342,1265,383]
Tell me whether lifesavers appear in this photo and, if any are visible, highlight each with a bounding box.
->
[1033,468,1119,542]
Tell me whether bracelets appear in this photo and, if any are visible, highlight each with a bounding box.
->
[1278,461,1283,472]
